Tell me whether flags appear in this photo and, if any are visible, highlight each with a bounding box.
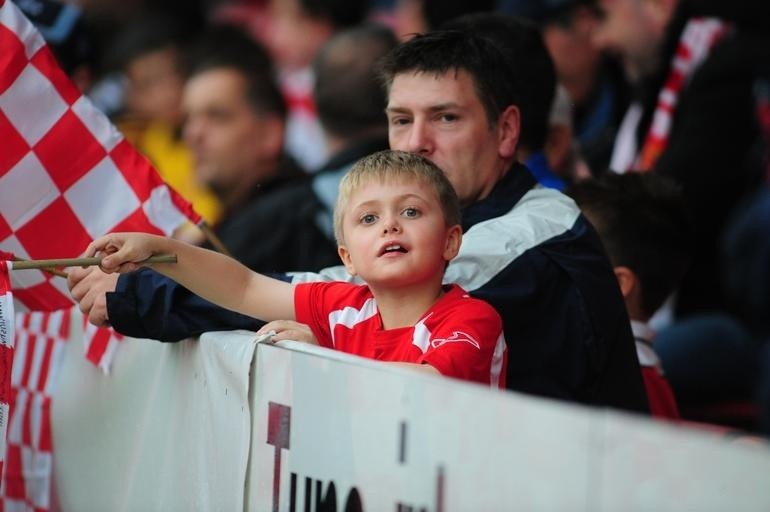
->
[1,303,63,510]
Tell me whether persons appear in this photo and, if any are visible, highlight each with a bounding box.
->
[1,0,769,443]
